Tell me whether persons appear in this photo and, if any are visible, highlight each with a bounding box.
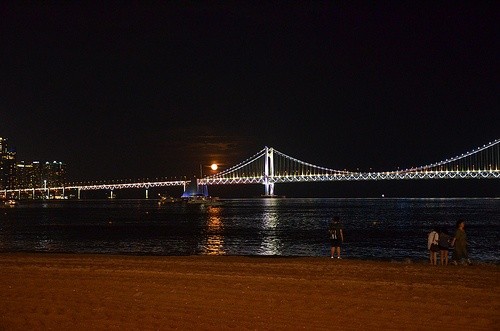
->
[451,219,472,265]
[428,225,439,264]
[439,224,450,266]
[328,216,344,260]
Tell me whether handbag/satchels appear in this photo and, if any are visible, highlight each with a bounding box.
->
[430,243,438,252]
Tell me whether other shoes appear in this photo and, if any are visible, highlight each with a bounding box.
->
[338,257,343,260]
[331,257,334,259]
